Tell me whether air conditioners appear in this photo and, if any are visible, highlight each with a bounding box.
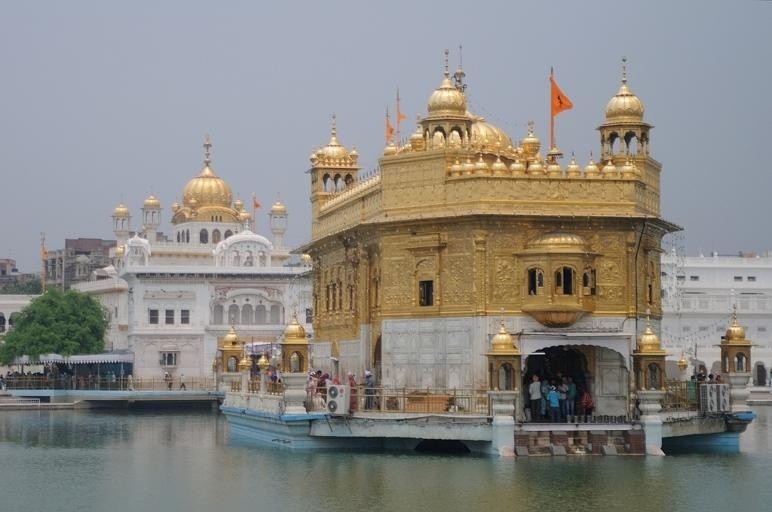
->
[325,382,351,416]
[714,382,730,413]
[699,382,719,415]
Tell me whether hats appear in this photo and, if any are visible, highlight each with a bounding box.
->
[347,371,372,378]
[531,374,538,381]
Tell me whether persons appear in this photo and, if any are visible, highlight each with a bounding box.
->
[305,368,378,411]
[523,373,596,425]
[1,358,282,396]
[690,373,723,386]
